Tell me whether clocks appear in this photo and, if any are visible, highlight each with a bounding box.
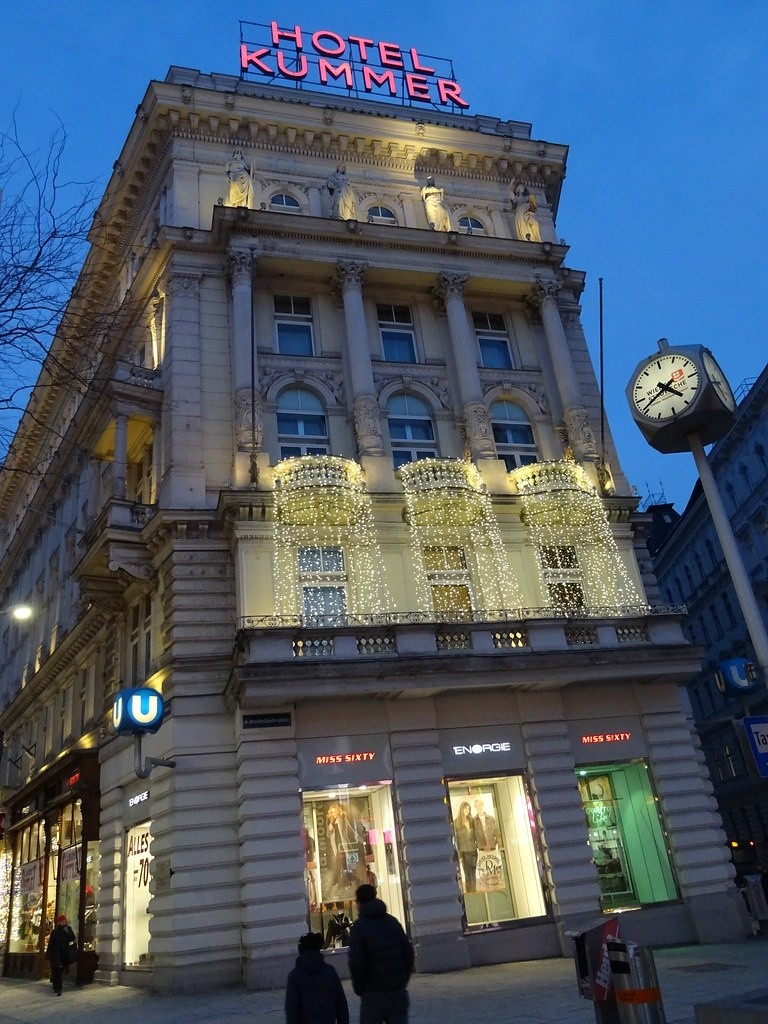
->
[626,342,740,453]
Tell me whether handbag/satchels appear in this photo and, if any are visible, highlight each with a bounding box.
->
[62,941,78,965]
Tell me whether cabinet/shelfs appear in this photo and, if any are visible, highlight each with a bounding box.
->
[583,799,634,896]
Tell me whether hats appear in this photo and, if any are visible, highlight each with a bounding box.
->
[54,914,71,926]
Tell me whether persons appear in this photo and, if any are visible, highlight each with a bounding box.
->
[325,805,366,890]
[46,915,76,996]
[473,799,499,851]
[285,932,349,1024]
[349,885,413,1024]
[454,802,479,892]
[324,911,354,949]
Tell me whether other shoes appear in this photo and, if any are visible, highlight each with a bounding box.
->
[53,983,63,996]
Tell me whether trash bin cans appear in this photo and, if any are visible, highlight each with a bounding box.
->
[605,938,667,1024]
